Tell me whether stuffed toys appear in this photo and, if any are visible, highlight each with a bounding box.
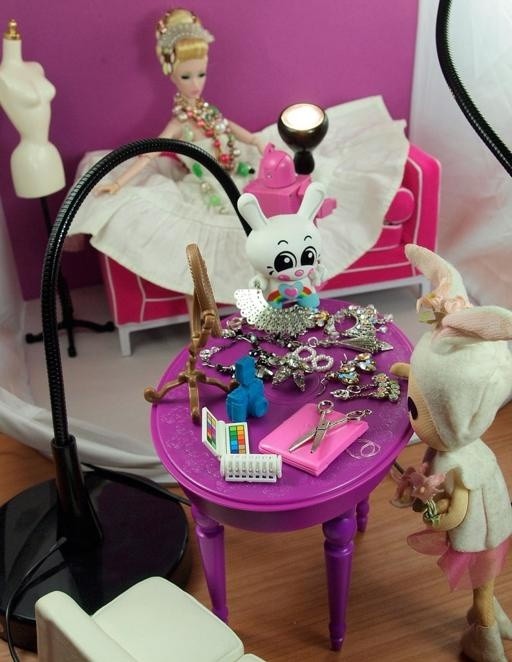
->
[389,243,512,662]
[238,181,325,308]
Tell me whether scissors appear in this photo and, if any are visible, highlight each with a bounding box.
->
[289,399,372,453]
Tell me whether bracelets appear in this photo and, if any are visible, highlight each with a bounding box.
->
[311,354,334,372]
[293,346,316,362]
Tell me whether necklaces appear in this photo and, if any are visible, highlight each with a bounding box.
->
[172,93,249,212]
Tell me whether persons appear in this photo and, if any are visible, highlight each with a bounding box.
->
[1,7,511,662]
[66,9,410,304]
[0,30,65,199]
